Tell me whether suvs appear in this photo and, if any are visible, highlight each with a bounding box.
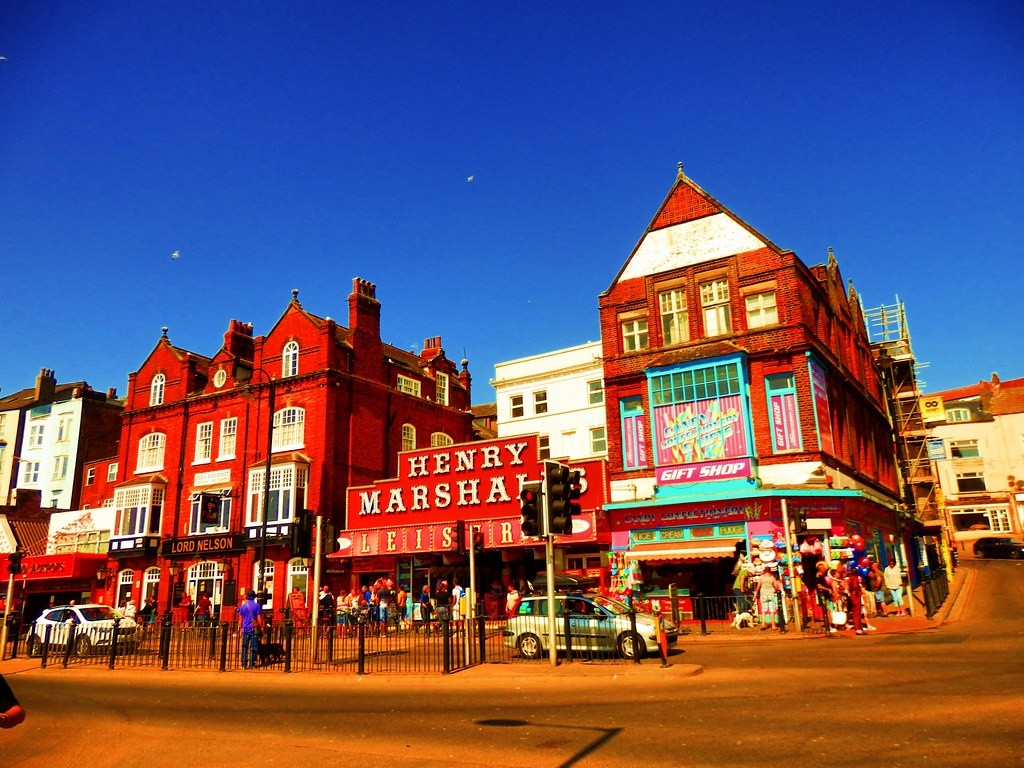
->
[26,605,143,657]
[973,537,1024,559]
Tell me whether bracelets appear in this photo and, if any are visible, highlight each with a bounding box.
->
[0,714,9,728]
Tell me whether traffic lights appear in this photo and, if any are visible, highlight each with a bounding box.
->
[793,507,809,545]
[520,489,541,537]
[474,533,485,554]
[8,551,21,575]
[544,459,582,536]
[452,520,466,557]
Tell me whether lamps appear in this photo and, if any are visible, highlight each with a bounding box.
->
[217,557,233,573]
[97,565,111,580]
[168,560,182,577]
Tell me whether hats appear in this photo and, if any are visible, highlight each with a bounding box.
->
[748,538,778,576]
[246,591,258,600]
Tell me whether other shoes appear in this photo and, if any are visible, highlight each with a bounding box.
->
[845,623,854,630]
[829,626,837,633]
[895,613,906,616]
[881,612,889,617]
[867,623,876,630]
[856,627,868,634]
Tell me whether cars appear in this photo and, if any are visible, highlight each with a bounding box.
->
[503,595,676,659]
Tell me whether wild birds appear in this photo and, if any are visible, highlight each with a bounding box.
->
[490,377,493,382]
[466,176,474,184]
[388,358,394,363]
[426,396,433,400]
[459,409,462,410]
[171,249,180,260]
[469,409,472,412]
[587,340,592,344]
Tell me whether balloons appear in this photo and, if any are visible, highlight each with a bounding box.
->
[851,535,865,550]
[857,557,870,567]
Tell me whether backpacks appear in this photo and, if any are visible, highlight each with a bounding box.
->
[455,587,465,604]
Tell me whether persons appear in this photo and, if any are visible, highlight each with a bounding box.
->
[884,559,906,616]
[70,600,76,605]
[237,590,266,670]
[413,585,433,638]
[569,600,602,619]
[434,578,464,638]
[120,591,214,631]
[733,566,779,630]
[505,583,521,616]
[817,558,888,634]
[66,609,82,625]
[0,673,26,729]
[294,580,411,637]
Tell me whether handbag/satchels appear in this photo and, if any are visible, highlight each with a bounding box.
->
[830,603,847,625]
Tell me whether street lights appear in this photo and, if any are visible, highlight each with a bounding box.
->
[242,368,275,613]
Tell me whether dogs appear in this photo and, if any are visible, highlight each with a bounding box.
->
[730,611,757,629]
[255,643,287,666]
